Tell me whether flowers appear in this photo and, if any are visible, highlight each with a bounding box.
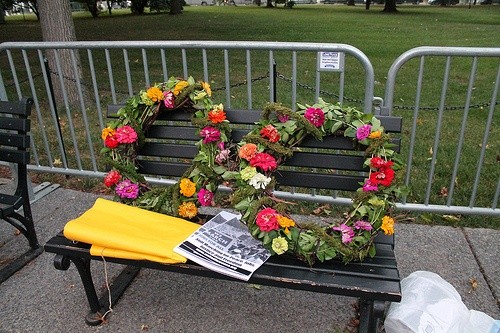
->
[235,98,398,267]
[100,72,233,218]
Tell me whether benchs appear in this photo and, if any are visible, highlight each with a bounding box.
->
[0,96,45,287]
[44,105,405,333]
